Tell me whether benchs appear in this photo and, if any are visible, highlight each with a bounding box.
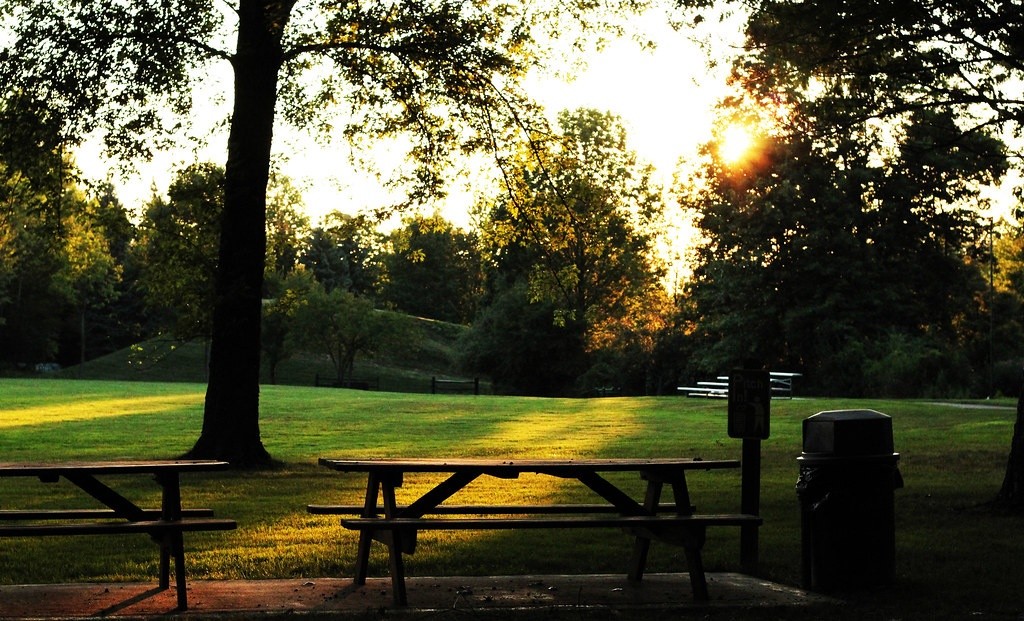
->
[0,518,237,557]
[306,501,697,535]
[0,507,215,542]
[341,512,763,556]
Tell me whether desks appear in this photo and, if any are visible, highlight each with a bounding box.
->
[317,457,740,606]
[0,460,230,612]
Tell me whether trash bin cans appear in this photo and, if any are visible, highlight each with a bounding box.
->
[794,408,901,600]
